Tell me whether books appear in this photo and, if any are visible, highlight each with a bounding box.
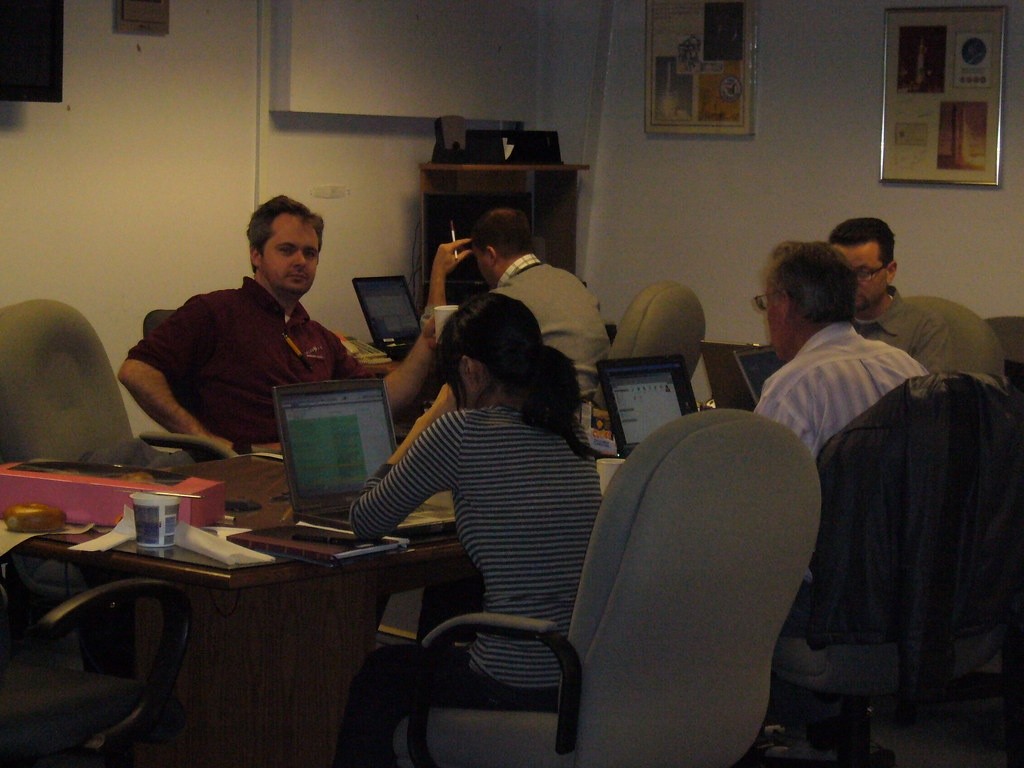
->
[227,522,412,568]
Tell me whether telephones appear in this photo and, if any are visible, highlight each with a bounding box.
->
[330,329,387,360]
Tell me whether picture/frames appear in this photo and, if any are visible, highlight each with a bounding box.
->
[879,5,1007,187]
[644,0,757,136]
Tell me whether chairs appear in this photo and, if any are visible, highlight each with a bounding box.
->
[982,316,1024,363]
[594,281,705,407]
[391,408,822,768]
[770,372,1024,768]
[0,300,239,470]
[0,553,192,768]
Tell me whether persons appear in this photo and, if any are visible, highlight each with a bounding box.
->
[118,196,445,451]
[335,292,604,768]
[422,208,611,398]
[751,217,955,640]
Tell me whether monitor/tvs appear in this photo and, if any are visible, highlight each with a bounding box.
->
[0,0,63,102]
[419,190,536,287]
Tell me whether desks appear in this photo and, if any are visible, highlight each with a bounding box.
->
[12,450,463,768]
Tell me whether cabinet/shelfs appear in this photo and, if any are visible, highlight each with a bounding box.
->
[418,162,590,300]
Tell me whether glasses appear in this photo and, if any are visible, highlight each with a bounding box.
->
[755,291,803,312]
[857,266,884,279]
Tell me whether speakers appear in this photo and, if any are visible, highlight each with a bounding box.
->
[432,115,467,163]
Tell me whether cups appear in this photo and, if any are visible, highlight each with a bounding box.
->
[433,305,458,344]
[130,492,180,547]
[596,459,626,497]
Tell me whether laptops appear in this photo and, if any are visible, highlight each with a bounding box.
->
[595,353,698,458]
[272,377,457,539]
[699,340,789,411]
[351,274,423,362]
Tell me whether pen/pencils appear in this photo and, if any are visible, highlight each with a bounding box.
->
[225,516,236,522]
[291,533,357,548]
[448,219,459,259]
[281,332,313,372]
